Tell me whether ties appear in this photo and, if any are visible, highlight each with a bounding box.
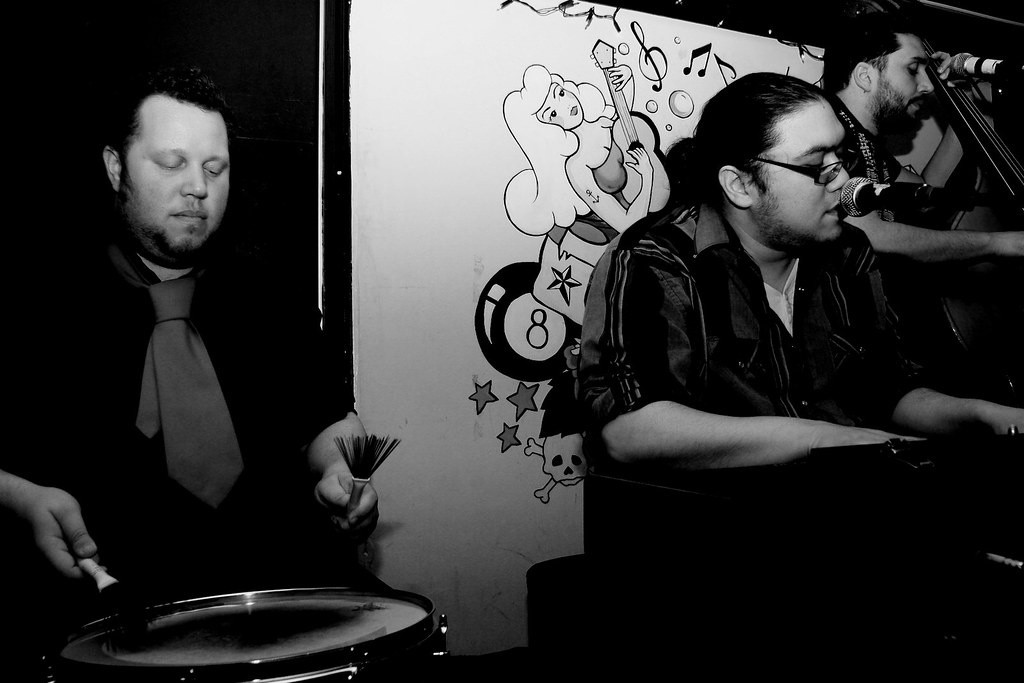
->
[107,246,245,508]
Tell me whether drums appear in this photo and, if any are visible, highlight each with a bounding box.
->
[47,584,449,683]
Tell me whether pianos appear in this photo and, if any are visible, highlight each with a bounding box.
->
[582,431,1024,683]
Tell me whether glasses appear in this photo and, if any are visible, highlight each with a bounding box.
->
[752,146,858,184]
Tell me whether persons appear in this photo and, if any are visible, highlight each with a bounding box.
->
[580,72,1017,473]
[1,64,378,683]
[820,15,1016,259]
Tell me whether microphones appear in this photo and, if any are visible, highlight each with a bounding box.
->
[949,53,1024,80]
[841,177,959,218]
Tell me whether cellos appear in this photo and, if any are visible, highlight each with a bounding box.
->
[863,0,1024,406]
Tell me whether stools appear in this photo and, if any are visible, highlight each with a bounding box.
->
[528,554,624,648]
